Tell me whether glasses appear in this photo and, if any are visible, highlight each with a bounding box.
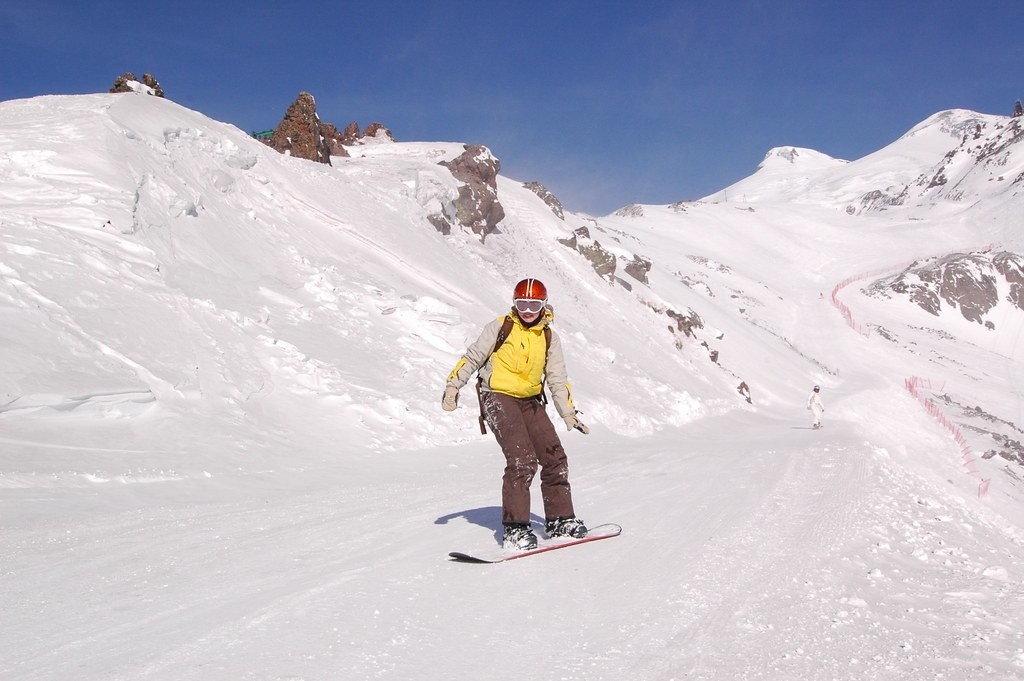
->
[513,299,543,313]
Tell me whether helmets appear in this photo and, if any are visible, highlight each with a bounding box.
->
[513,278,548,307]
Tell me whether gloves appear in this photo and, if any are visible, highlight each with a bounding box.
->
[562,413,591,434]
[441,386,459,411]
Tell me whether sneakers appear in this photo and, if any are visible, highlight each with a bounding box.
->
[502,524,538,551]
[544,515,588,539]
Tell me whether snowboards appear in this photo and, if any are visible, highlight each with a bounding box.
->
[448,522,623,564]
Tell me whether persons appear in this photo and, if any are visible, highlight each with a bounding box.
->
[442,278,590,551]
[807,385,825,428]
